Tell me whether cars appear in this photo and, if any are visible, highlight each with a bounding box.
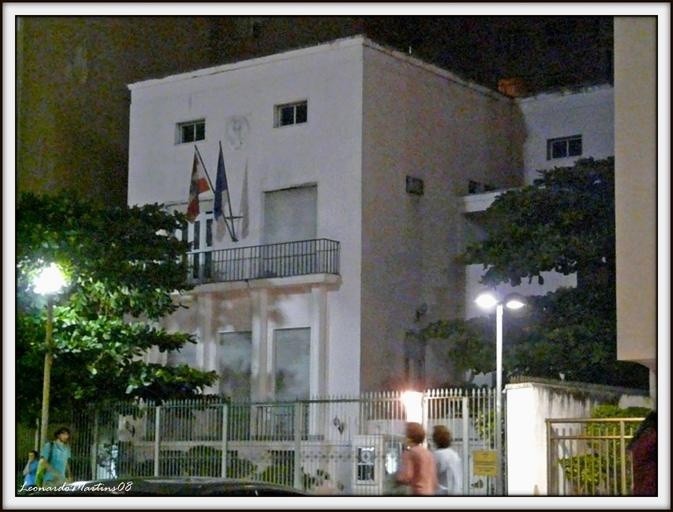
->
[72,477,307,496]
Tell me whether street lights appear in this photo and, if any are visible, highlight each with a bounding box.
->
[476,294,524,496]
[33,264,65,452]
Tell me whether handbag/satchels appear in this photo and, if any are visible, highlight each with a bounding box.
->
[35,442,53,488]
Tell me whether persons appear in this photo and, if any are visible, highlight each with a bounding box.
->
[394,422,439,496]
[22,449,39,489]
[39,427,74,488]
[431,424,464,495]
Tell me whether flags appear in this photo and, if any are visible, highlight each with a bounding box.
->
[213,144,228,221]
[185,149,212,224]
